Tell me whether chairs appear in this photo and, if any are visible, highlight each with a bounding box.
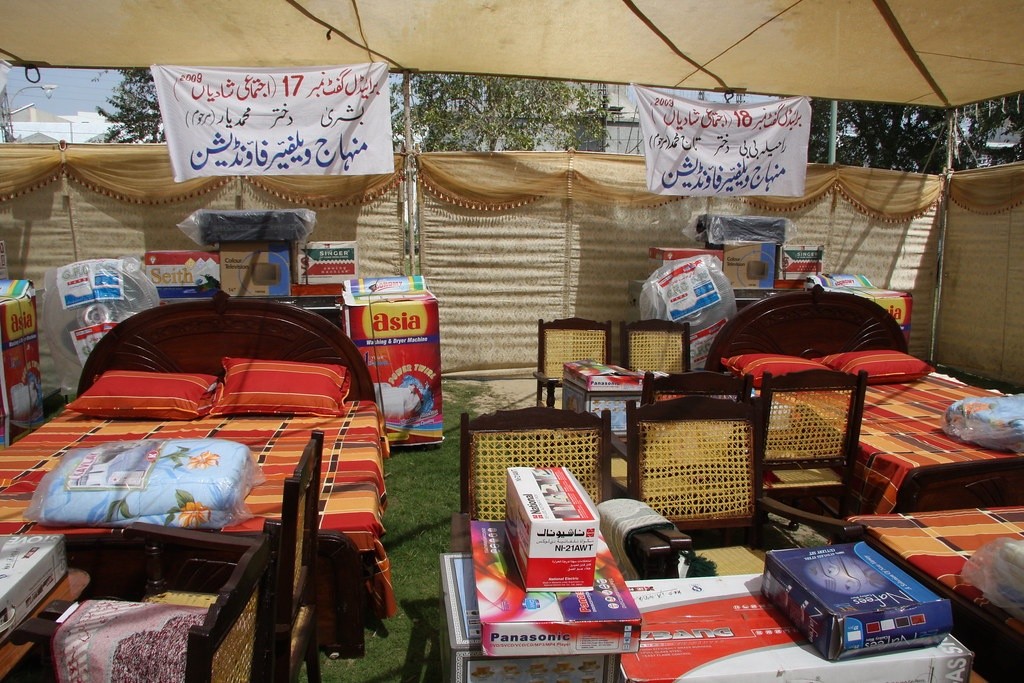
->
[449,405,613,553]
[10,427,324,683]
[619,319,691,373]
[532,317,612,464]
[611,368,869,578]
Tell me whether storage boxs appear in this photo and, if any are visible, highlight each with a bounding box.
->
[0,533,68,644]
[438,466,975,682]
[774,244,913,346]
[561,358,798,436]
[705,241,776,288]
[144,238,445,447]
[0,278,43,449]
[647,247,723,279]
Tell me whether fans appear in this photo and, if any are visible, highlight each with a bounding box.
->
[43,258,157,365]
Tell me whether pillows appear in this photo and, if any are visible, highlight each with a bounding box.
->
[721,353,832,388]
[65,370,219,420]
[208,355,353,418]
[812,349,935,385]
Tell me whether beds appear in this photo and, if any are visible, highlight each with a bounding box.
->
[0,289,397,659]
[701,285,1023,512]
[828,504,1024,683]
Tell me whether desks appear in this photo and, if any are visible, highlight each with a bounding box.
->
[734,288,807,304]
[227,294,345,329]
[0,566,91,683]
